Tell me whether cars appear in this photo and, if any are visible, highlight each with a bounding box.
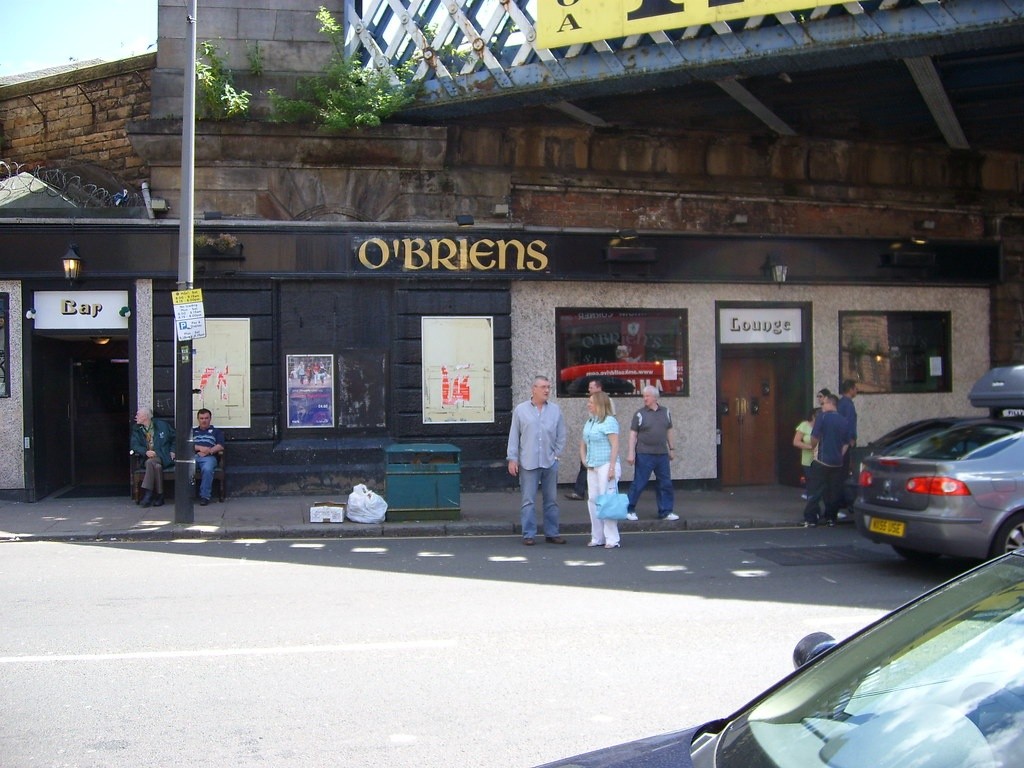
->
[851,365,1024,556]
[866,416,963,452]
[540,543,1024,768]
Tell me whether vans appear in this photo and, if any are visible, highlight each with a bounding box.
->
[560,359,685,395]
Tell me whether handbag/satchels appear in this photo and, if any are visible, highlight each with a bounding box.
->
[595,478,629,521]
[346,483,389,524]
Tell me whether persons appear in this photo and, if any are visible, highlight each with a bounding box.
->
[297,360,327,385]
[626,385,680,521]
[792,379,858,528]
[579,391,621,548]
[565,379,616,499]
[131,406,177,507]
[616,345,634,362]
[192,409,225,506]
[505,375,567,545]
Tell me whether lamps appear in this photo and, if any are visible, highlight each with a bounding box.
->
[911,234,931,244]
[772,259,791,287]
[606,228,639,246]
[152,198,170,213]
[59,239,83,286]
[203,209,220,219]
[733,213,749,224]
[921,220,935,229]
[89,337,112,346]
[454,214,476,226]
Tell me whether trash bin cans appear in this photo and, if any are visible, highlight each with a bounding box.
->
[382,443,461,521]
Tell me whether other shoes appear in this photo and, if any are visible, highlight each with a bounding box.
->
[139,500,151,508]
[627,512,639,520]
[587,541,598,547]
[604,542,621,548]
[545,536,566,544]
[662,512,680,521]
[836,511,848,519]
[564,492,585,500]
[153,494,165,506]
[823,520,835,527]
[800,520,817,527]
[524,538,536,545]
[800,490,807,500]
[200,498,208,506]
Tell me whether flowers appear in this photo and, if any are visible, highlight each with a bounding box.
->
[195,231,239,251]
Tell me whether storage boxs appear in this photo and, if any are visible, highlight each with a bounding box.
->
[491,201,513,216]
[308,500,346,524]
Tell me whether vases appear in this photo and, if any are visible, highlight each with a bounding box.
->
[195,243,243,260]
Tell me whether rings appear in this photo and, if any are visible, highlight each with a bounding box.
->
[608,478,611,480]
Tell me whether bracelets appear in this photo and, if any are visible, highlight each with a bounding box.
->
[670,449,675,450]
[609,468,615,471]
[554,456,561,461]
[211,452,213,456]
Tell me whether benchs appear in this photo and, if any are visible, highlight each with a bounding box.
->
[132,446,226,505]
